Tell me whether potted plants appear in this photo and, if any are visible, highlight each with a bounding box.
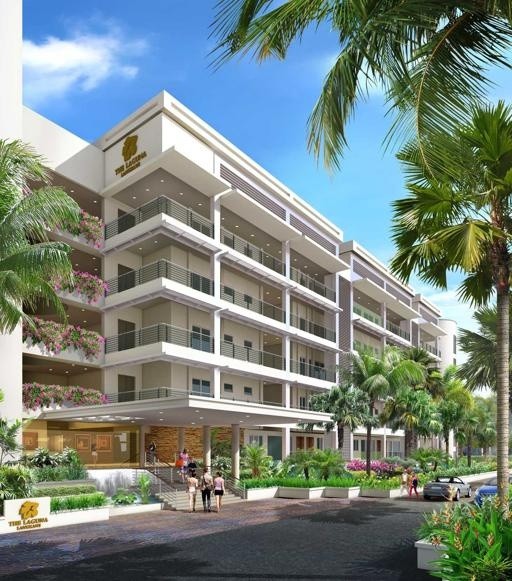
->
[137,472,153,503]
[111,492,137,505]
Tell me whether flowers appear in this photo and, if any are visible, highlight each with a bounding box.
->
[413,483,475,550]
[48,267,109,305]
[22,382,111,415]
[58,204,105,249]
[23,316,105,360]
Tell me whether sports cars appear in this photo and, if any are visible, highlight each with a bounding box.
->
[423,473,473,502]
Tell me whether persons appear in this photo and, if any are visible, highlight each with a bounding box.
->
[399,469,410,497]
[408,471,419,497]
[214,472,225,512]
[91,443,98,464]
[149,440,156,459]
[178,447,197,483]
[200,467,213,512]
[186,472,199,513]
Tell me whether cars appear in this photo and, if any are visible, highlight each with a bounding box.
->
[474,475,512,506]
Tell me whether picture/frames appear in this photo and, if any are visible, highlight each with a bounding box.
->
[75,434,91,453]
[22,431,38,451]
[96,435,112,453]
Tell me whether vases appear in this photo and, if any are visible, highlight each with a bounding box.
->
[415,535,452,571]
